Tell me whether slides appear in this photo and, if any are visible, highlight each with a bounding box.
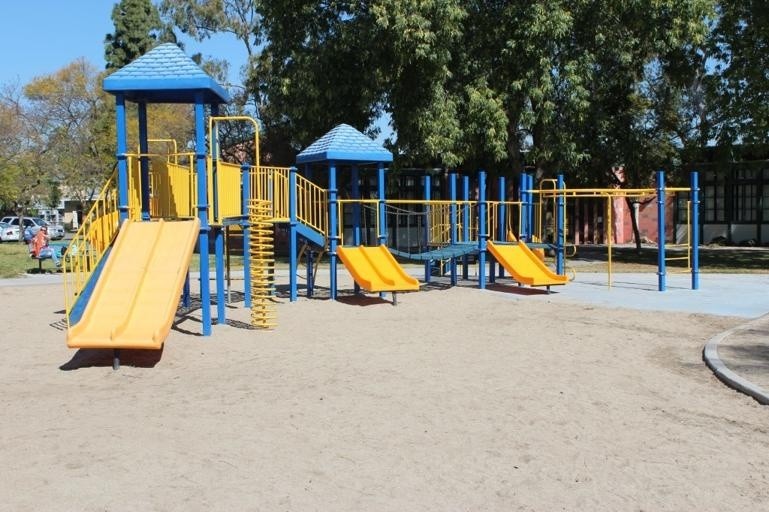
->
[487,240,568,286]
[66,217,201,349]
[337,244,419,292]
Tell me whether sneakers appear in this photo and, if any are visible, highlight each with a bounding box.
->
[56,257,70,267]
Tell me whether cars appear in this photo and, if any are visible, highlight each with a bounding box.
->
[0,222,25,243]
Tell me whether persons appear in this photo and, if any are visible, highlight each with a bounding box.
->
[23,224,34,241]
[31,226,68,267]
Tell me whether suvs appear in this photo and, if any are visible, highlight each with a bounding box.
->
[0,216,66,241]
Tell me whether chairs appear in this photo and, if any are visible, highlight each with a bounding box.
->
[29,240,97,273]
